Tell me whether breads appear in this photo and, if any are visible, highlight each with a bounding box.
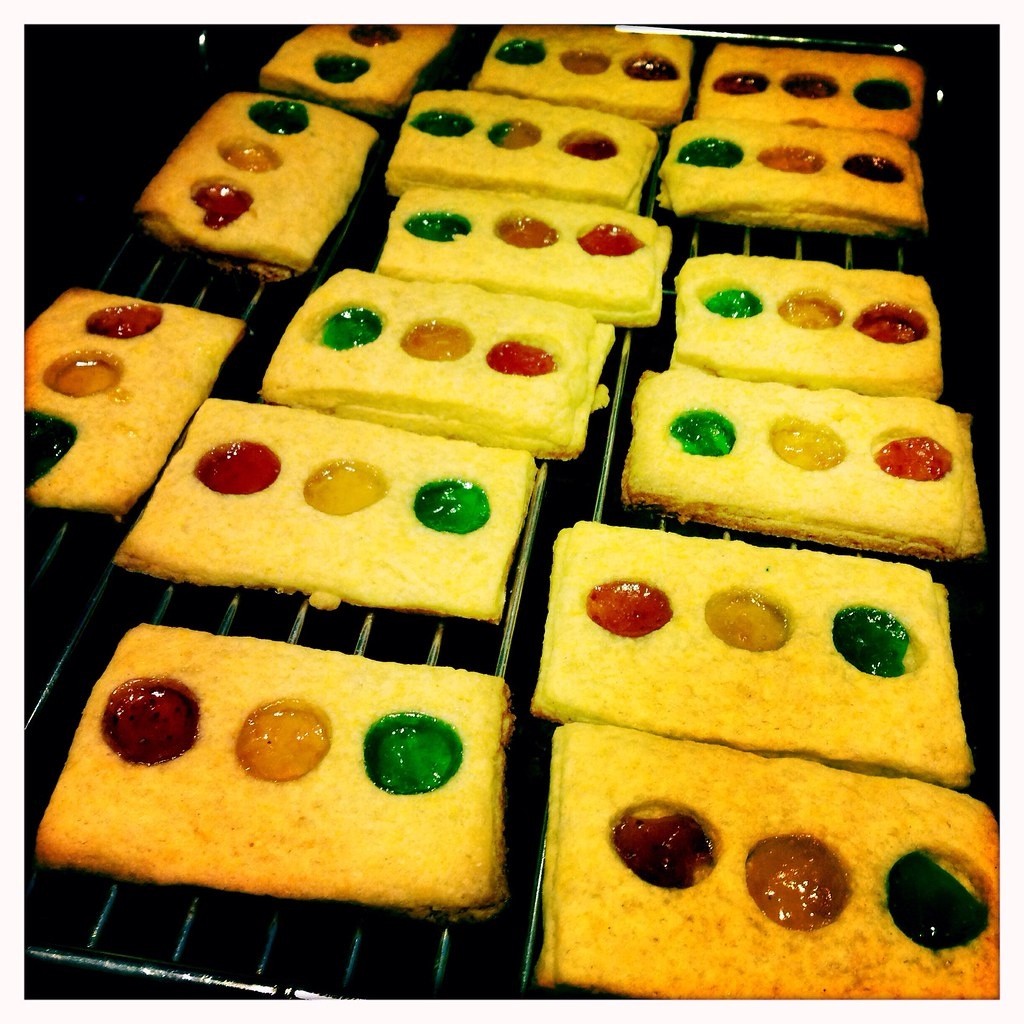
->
[26,23,999,999]
